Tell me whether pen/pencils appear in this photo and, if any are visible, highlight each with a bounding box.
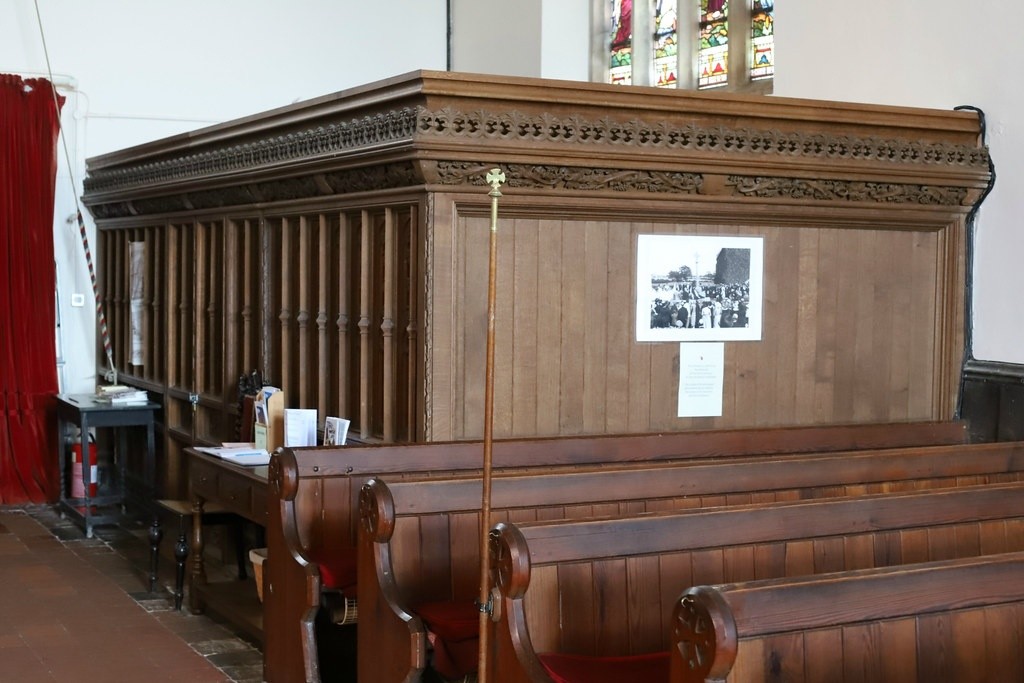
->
[215,447,232,449]
[69,398,78,403]
[236,453,261,456]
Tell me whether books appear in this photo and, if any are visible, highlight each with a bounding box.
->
[284,409,350,446]
[95,385,149,405]
[201,447,271,466]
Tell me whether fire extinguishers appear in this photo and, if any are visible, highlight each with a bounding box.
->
[70,424,98,517]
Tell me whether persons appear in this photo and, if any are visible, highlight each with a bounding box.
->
[651,279,749,329]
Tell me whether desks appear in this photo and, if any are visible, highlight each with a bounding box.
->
[54,392,160,539]
[179,445,271,614]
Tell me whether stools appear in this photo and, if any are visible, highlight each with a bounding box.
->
[147,497,248,613]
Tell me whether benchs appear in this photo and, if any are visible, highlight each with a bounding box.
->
[259,419,1024,683]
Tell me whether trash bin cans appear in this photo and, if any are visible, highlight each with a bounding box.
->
[250,548,267,603]
